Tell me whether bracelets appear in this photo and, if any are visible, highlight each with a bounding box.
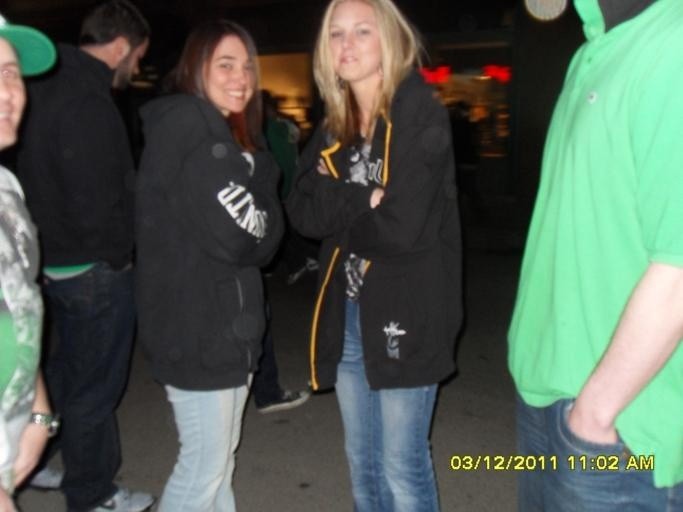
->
[28,414,57,439]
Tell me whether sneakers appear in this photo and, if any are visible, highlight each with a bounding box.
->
[255,390,311,415]
[26,466,64,489]
[89,488,154,512]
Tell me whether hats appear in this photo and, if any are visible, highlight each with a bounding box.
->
[0,15,58,77]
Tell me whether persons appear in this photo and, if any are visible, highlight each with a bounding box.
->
[501,0,681,512]
[0,11,58,512]
[280,0,465,512]
[254,84,302,209]
[12,3,157,511]
[131,16,286,512]
[253,279,312,418]
